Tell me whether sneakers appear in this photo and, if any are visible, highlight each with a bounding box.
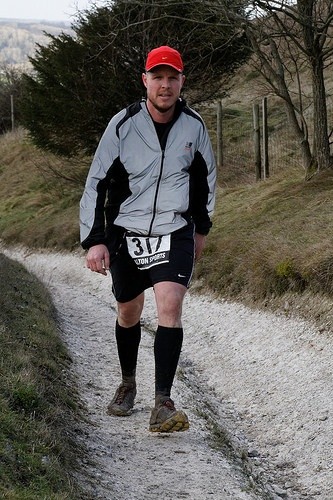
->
[149,399,189,432]
[107,381,137,415]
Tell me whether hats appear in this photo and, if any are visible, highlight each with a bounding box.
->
[145,46,184,74]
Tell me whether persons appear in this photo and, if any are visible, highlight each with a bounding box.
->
[76,47,217,433]
